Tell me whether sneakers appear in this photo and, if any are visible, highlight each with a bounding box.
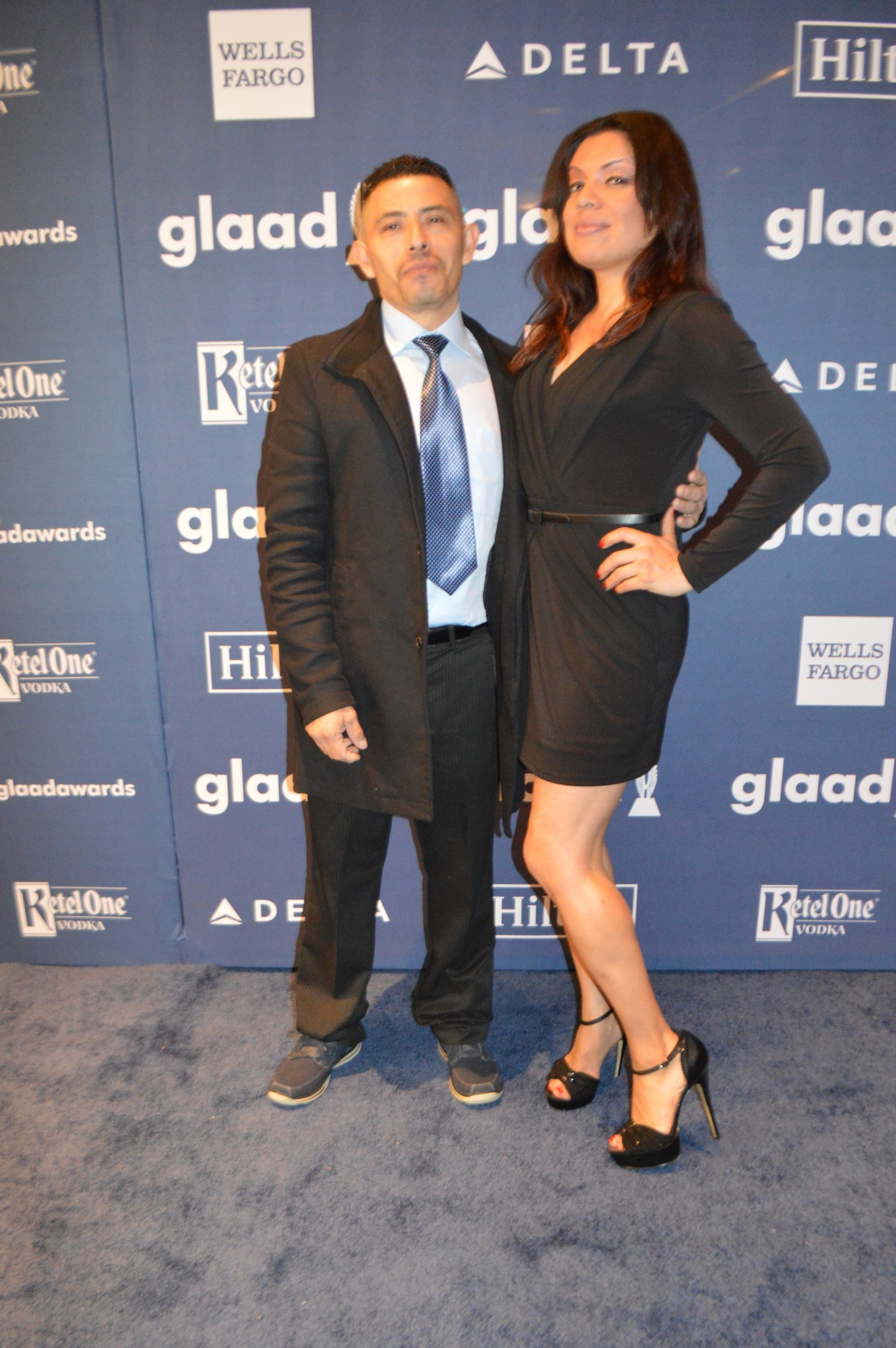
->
[438,1036,505,1104]
[264,1030,363,1106]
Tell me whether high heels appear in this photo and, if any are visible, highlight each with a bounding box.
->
[545,1006,630,1110]
[605,1030,719,1169]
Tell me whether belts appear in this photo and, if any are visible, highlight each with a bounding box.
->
[528,506,687,526]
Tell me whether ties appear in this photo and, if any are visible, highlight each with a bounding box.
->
[411,332,478,597]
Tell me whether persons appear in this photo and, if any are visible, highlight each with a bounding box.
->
[248,156,710,1115]
[495,108,835,1173]
